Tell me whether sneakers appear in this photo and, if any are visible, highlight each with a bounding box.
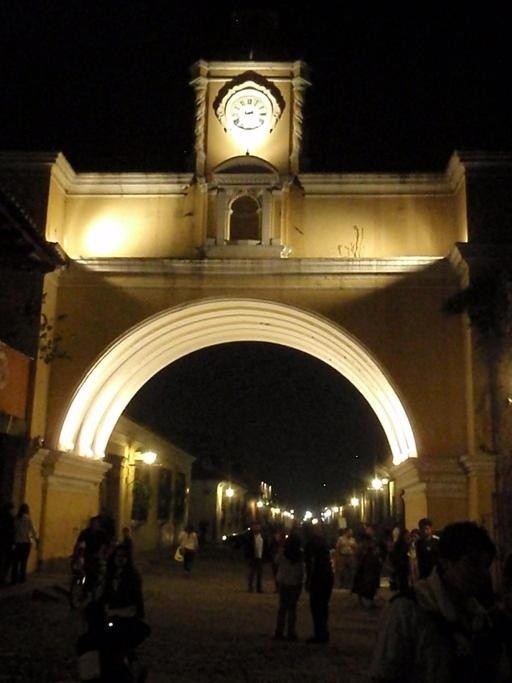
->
[284,631,302,642]
[269,630,289,642]
[306,633,331,645]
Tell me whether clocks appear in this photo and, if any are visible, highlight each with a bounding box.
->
[214,78,283,141]
